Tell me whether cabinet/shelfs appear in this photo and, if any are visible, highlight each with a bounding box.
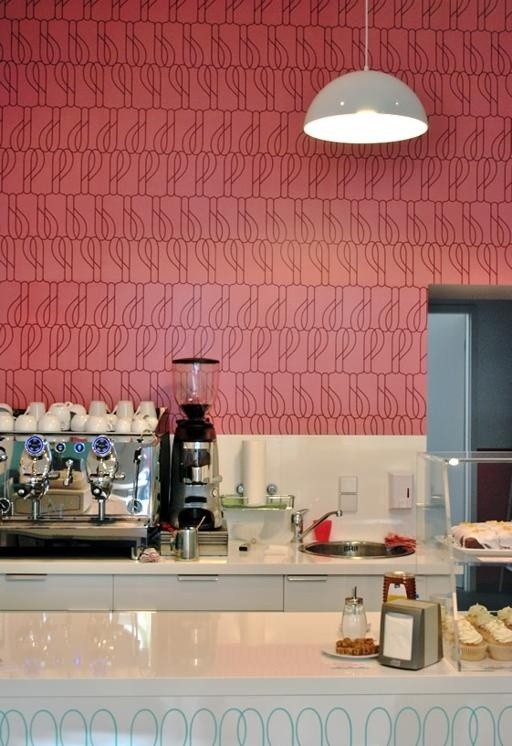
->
[1,555,111,614]
[403,443,512,677]
[273,558,457,610]
[102,551,286,612]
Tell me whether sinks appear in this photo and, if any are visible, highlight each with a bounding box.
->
[298,540,415,558]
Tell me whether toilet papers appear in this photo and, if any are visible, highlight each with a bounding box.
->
[241,440,266,508]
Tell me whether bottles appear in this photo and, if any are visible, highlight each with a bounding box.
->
[342,597,366,638]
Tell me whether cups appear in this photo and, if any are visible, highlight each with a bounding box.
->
[311,519,333,544]
[0,397,160,434]
[171,528,201,561]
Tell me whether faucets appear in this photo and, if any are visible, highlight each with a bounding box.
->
[291,508,342,543]
[63,459,74,486]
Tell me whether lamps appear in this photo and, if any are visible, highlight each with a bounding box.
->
[299,0,433,149]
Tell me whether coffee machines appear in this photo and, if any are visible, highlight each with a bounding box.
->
[168,358,225,527]
[1,436,162,560]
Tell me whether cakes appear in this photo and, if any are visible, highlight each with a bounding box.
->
[442,602,512,663]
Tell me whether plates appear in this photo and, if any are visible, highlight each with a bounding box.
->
[437,535,511,557]
[320,648,379,660]
